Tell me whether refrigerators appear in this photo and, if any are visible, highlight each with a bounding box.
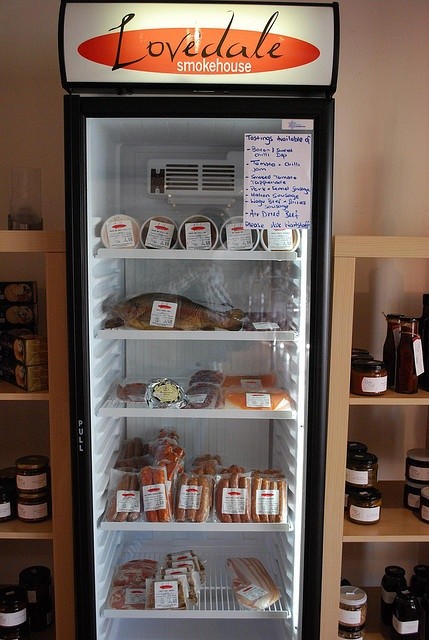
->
[58,0,341,639]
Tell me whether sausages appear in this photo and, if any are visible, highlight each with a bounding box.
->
[106,431,287,525]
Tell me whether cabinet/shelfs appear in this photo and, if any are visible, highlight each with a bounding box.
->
[1,229,75,638]
[317,234,428,636]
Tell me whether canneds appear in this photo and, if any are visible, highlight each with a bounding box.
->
[339,584,367,639]
[347,442,383,525]
[16,493,51,522]
[19,565,51,602]
[14,457,52,490]
[1,485,15,522]
[400,446,429,523]
[353,359,388,397]
[377,561,429,631]
[1,584,29,640]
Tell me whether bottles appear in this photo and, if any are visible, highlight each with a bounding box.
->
[381,314,405,391]
[408,295,428,392]
[394,315,420,395]
